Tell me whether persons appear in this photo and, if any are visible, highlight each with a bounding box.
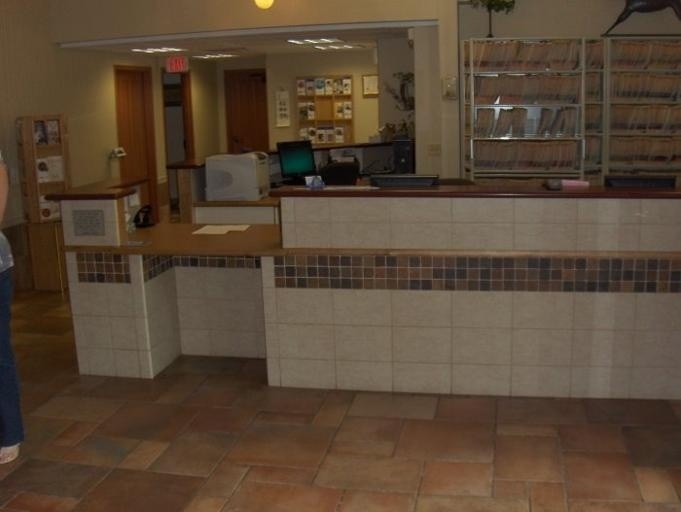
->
[0,154,28,464]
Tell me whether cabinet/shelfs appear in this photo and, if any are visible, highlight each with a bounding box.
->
[297,74,356,145]
[14,111,71,221]
[463,31,681,181]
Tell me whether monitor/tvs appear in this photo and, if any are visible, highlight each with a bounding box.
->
[277,140,317,185]
[604,176,676,188]
[369,174,439,187]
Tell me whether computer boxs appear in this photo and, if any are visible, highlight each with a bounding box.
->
[205,151,271,202]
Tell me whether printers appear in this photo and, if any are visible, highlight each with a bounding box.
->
[319,154,361,185]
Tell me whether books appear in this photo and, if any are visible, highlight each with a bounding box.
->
[298,78,352,145]
